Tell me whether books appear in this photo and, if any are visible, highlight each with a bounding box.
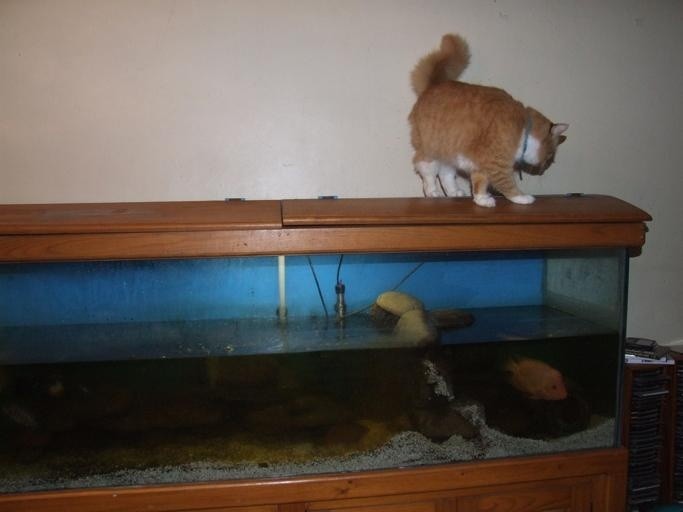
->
[625,334,683,364]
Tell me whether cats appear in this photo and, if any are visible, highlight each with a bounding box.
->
[407,34,569,209]
